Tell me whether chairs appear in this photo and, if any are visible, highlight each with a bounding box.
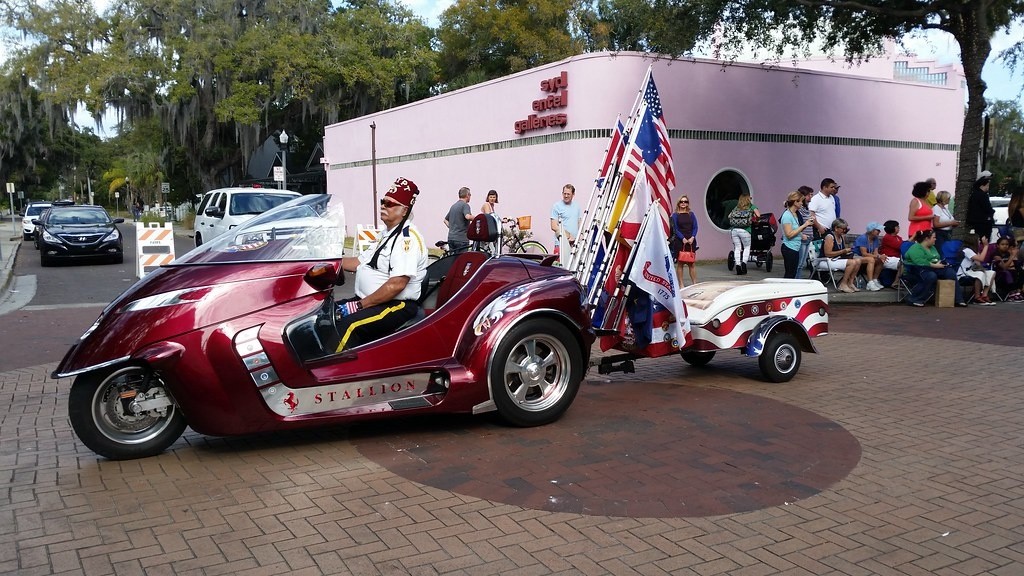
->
[808,235,1018,304]
[396,213,499,332]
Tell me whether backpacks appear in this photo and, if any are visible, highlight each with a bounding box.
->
[730,204,752,227]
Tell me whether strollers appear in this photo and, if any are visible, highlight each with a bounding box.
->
[728,212,779,272]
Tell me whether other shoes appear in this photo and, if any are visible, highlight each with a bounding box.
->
[736,263,747,275]
[892,282,906,290]
[838,283,861,293]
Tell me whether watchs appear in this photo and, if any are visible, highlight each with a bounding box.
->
[356,300,363,311]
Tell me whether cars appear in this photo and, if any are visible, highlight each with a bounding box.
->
[31,199,125,267]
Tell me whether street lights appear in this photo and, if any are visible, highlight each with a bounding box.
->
[279,129,288,190]
[125,177,130,209]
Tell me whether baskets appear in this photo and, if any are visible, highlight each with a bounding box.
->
[516,216,531,229]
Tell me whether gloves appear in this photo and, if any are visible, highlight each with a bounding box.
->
[337,300,363,318]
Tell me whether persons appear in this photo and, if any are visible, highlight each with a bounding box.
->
[132,201,140,222]
[672,196,699,290]
[314,176,428,354]
[781,176,1024,307]
[551,184,583,262]
[444,187,507,256]
[728,195,761,275]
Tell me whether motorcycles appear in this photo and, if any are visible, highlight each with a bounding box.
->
[50,192,596,460]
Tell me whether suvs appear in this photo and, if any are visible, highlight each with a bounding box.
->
[19,202,52,241]
[193,186,344,256]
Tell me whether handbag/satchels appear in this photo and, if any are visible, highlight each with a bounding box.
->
[678,243,696,264]
[997,217,1013,237]
[840,251,854,259]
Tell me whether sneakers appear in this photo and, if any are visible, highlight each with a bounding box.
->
[973,296,996,305]
[1006,293,1024,302]
[866,281,884,292]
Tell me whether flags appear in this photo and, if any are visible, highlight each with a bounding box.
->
[569,72,690,352]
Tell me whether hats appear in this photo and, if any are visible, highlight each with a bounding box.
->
[834,183,841,189]
[383,177,419,208]
[979,170,992,177]
[864,222,886,233]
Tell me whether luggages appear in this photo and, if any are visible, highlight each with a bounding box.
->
[756,212,778,234]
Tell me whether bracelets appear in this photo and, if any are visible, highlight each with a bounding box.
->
[877,255,880,259]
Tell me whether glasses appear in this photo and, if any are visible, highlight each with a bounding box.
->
[928,230,934,238]
[895,226,899,228]
[468,194,471,196]
[680,200,689,204]
[838,226,850,232]
[876,229,880,232]
[380,198,403,208]
[926,183,950,199]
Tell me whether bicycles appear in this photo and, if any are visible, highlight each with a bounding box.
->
[471,213,548,255]
[427,238,493,265]
